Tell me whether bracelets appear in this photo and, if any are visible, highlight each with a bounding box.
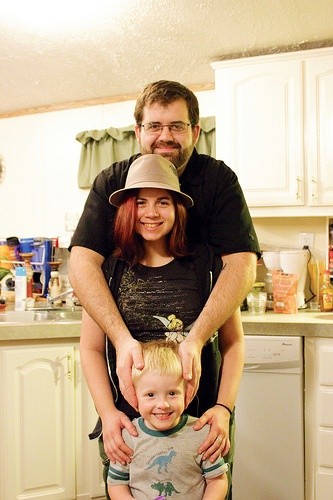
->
[215,403,233,417]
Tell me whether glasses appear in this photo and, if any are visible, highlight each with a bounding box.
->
[139,123,194,135]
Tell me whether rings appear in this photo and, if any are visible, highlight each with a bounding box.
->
[217,438,223,443]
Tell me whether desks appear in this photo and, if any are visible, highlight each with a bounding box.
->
[0,310,332,500]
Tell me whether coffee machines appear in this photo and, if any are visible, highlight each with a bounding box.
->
[262,250,307,311]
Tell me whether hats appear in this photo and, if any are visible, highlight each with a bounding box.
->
[109,154,194,208]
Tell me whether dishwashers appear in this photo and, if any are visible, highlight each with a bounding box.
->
[233,335,305,491]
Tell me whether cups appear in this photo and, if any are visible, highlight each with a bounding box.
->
[247,292,267,315]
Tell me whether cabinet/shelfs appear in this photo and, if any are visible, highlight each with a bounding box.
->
[210,45,333,216]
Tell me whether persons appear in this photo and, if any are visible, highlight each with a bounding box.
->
[66,80,276,492]
[103,339,229,500]
[77,153,246,500]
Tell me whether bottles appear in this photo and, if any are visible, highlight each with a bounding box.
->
[319,271,333,312]
[48,271,62,300]
